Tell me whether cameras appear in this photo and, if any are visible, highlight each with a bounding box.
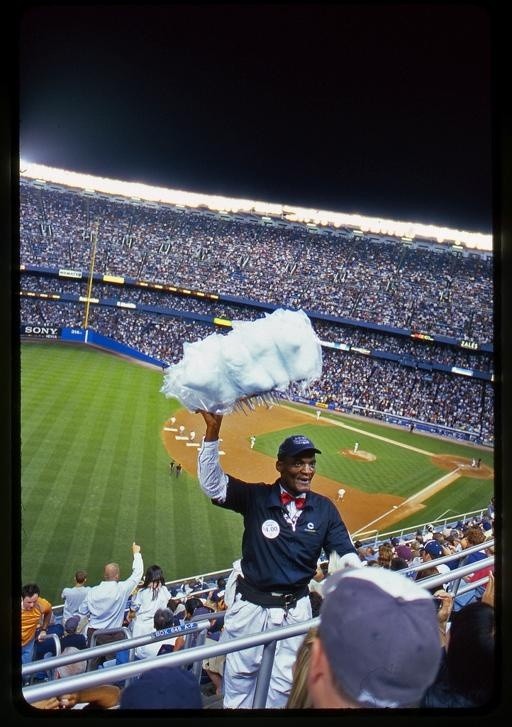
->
[433,599,443,614]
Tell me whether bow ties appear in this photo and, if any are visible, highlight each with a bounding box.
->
[279,490,306,511]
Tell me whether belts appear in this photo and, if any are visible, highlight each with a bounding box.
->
[237,574,307,609]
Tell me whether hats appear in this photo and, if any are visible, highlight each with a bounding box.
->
[117,664,208,710]
[417,538,443,556]
[276,434,322,456]
[395,544,413,562]
[64,615,81,632]
[317,564,450,708]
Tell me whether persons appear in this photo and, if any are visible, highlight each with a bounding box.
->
[336,488,346,503]
[170,460,182,477]
[197,408,363,710]
[354,441,360,454]
[472,457,482,468]
[21,497,496,709]
[20,182,494,443]
[250,436,256,449]
